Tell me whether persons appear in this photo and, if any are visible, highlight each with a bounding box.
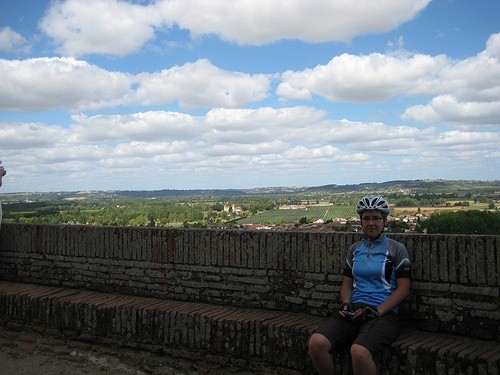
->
[309,195,410,375]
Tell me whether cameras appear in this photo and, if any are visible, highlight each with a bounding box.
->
[342,309,356,316]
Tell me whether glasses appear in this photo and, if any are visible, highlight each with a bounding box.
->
[362,216,383,221]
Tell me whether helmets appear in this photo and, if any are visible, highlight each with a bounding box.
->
[356,195,389,215]
